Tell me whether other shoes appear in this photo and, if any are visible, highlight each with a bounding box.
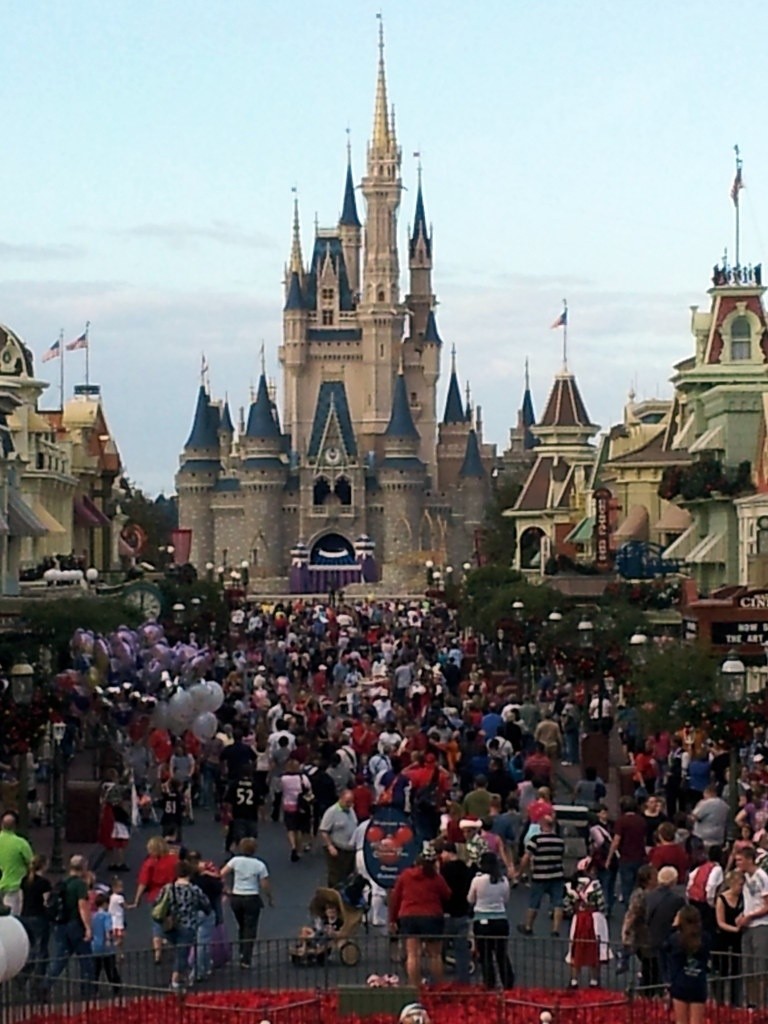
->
[237,952,252,970]
[588,984,600,989]
[290,848,300,863]
[566,980,579,990]
[372,918,387,928]
[510,879,519,890]
[550,931,561,943]
[517,923,533,936]
[171,981,186,990]
[107,862,130,873]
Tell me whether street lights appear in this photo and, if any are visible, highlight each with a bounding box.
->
[720,649,746,842]
[424,558,443,593]
[577,614,594,723]
[45,722,67,873]
[512,598,533,695]
[10,662,35,840]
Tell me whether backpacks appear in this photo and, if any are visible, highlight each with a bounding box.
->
[48,875,80,926]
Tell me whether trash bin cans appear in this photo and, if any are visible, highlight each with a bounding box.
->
[550,806,589,878]
[62,779,104,845]
[579,731,609,783]
[617,766,639,819]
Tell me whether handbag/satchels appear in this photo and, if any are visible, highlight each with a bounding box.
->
[151,882,177,933]
[111,821,130,839]
[589,827,618,875]
[295,774,315,818]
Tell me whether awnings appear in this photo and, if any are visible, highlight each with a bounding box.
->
[562,517,596,543]
[655,504,692,533]
[662,527,726,563]
[8,493,68,537]
[74,494,113,528]
[612,503,649,542]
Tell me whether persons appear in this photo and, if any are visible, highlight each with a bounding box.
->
[0,701,178,1001]
[156,585,767,1024]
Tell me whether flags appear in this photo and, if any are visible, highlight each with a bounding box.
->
[731,162,746,204]
[551,312,566,327]
[201,356,208,374]
[43,340,60,362]
[66,334,86,350]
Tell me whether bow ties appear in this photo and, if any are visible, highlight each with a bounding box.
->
[343,809,350,814]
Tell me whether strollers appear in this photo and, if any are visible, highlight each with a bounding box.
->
[289,886,365,968]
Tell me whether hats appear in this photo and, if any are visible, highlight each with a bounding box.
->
[459,815,483,829]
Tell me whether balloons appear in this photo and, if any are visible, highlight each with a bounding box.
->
[51,621,223,781]
